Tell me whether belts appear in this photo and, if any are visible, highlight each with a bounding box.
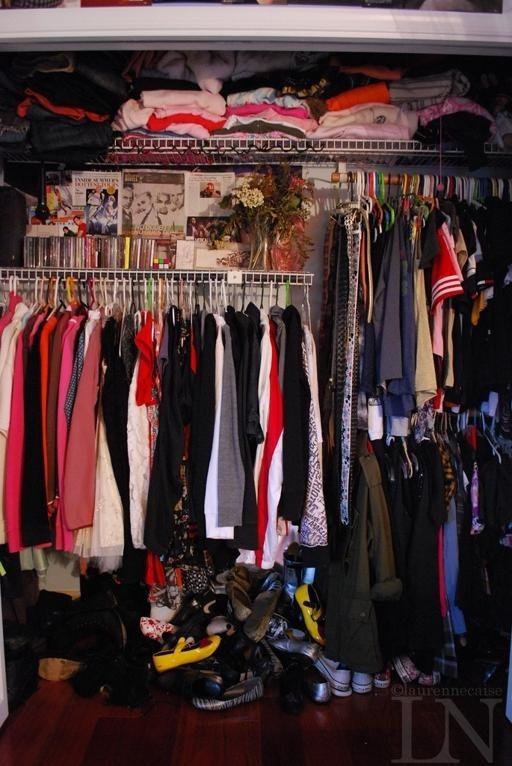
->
[313,206,362,527]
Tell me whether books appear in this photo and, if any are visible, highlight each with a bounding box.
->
[24,236,157,270]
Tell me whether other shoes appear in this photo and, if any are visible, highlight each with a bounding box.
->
[1,545,352,711]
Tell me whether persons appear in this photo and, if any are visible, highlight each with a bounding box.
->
[31,183,185,236]
[187,217,218,237]
[200,182,220,198]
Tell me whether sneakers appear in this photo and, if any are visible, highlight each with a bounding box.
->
[353,655,440,693]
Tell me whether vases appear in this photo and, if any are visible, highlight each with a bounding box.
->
[247,223,274,271]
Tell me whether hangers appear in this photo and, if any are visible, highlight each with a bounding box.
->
[346,169,512,243]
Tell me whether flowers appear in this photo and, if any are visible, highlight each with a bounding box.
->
[218,157,315,243]
